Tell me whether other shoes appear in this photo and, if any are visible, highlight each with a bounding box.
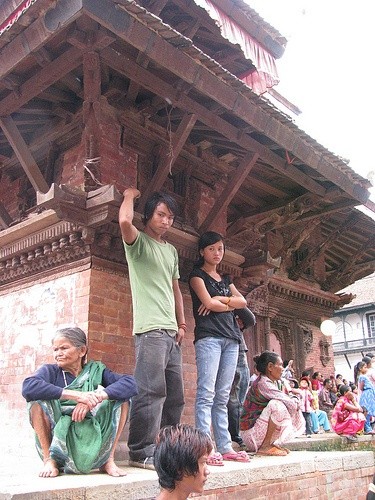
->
[204,452,224,466]
[129,456,155,470]
[222,451,251,462]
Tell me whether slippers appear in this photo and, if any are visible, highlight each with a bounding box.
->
[256,446,287,456]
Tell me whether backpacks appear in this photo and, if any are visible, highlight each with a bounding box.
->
[235,306,256,331]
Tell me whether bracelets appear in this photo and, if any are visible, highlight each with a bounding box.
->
[225,305,231,311]
[226,297,232,303]
[179,323,188,331]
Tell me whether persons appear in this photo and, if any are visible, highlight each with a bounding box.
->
[21,327,139,477]
[190,231,252,466]
[242,353,375,441]
[239,352,301,456]
[151,424,213,500]
[119,186,187,468]
